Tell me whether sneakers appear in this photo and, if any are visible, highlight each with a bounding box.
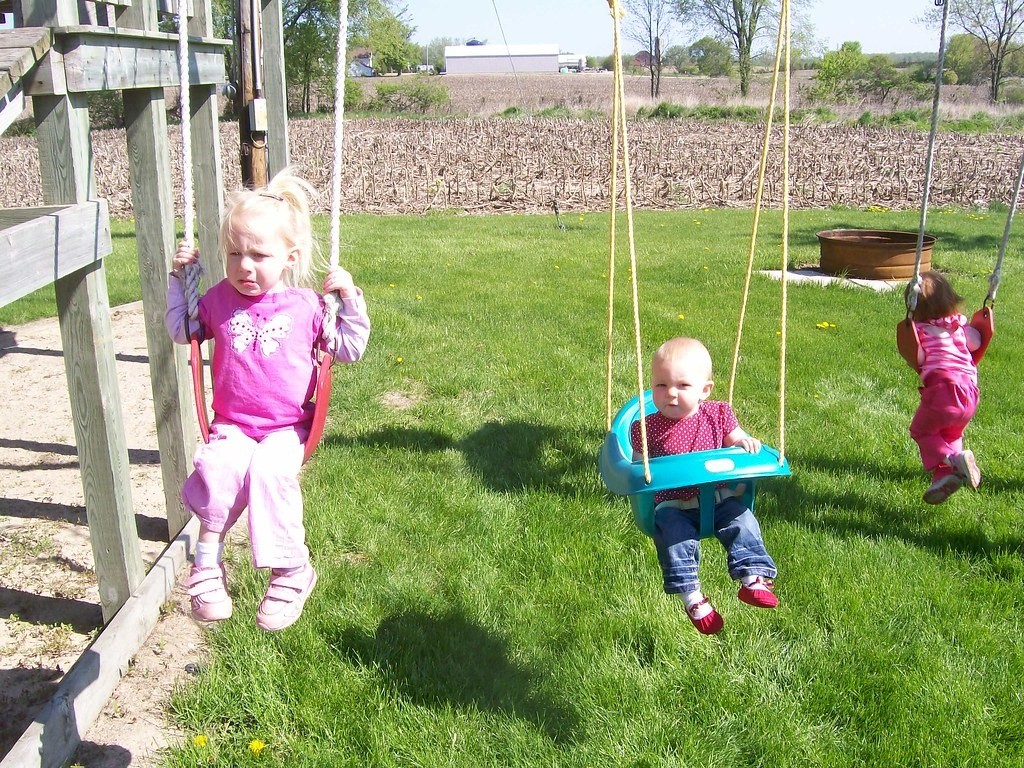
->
[948,450,980,488]
[923,467,961,505]
[184,562,232,622]
[256,561,317,630]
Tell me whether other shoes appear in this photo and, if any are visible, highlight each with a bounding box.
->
[685,597,723,634]
[738,576,777,608]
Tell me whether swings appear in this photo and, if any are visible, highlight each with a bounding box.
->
[599,0,795,541]
[177,0,349,468]
[895,0,1024,377]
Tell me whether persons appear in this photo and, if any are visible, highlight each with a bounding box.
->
[630,335,777,634]
[904,271,980,504]
[164,163,371,631]
